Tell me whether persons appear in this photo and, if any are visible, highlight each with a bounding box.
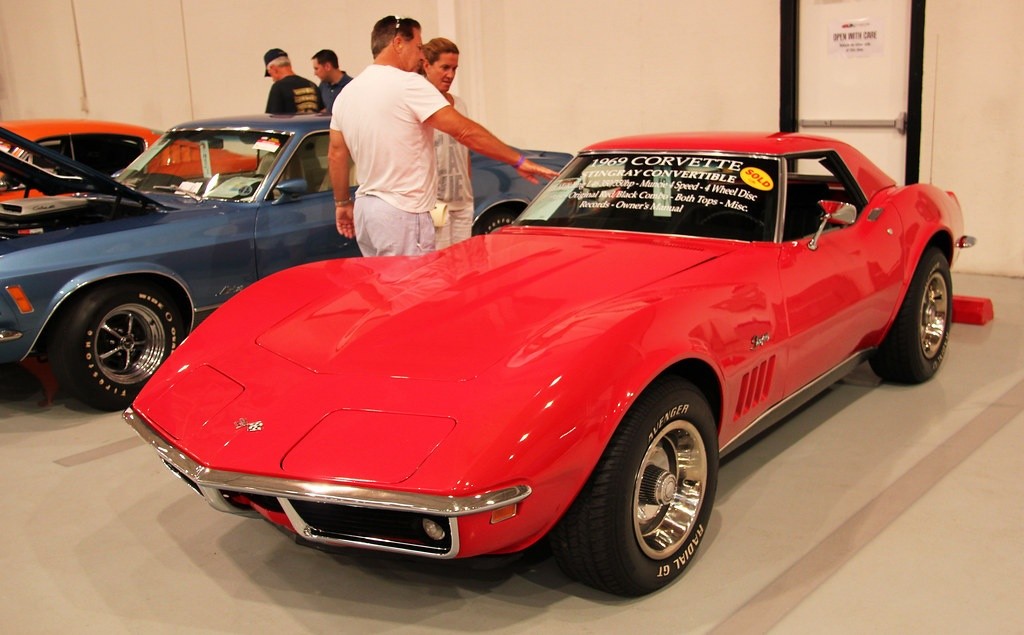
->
[312,49,353,115]
[328,16,561,258]
[417,38,474,251]
[264,48,327,114]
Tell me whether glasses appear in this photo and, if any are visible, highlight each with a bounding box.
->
[394,16,404,39]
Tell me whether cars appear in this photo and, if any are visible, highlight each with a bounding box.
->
[1,118,575,414]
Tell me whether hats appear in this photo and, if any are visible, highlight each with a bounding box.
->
[264,48,287,77]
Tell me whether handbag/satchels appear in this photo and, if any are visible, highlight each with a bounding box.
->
[429,203,450,227]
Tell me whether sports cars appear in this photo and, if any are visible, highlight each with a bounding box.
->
[123,133,962,599]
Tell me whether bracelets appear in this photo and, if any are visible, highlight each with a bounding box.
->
[512,156,526,168]
[335,197,352,206]
